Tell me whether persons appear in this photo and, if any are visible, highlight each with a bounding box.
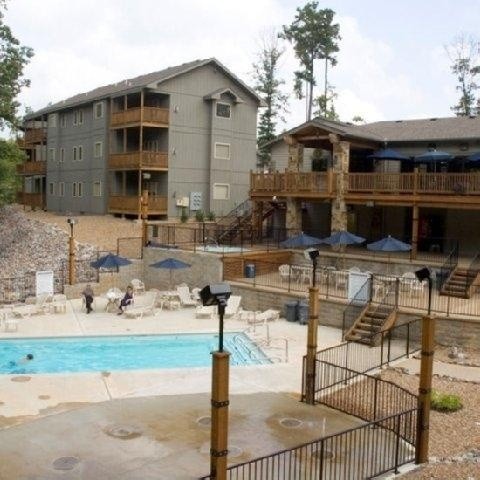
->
[413,263,436,289]
[192,285,201,308]
[116,284,133,316]
[0,353,33,371]
[79,284,94,314]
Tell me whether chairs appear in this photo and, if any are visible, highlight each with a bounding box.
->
[1,277,280,330]
[279,261,426,303]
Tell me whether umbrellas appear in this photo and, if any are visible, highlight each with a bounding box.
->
[366,234,412,274]
[322,229,367,268]
[365,147,480,174]
[86,252,132,273]
[148,257,192,290]
[278,230,327,250]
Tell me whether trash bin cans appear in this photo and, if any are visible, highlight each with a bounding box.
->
[286,300,309,324]
[246,265,256,279]
